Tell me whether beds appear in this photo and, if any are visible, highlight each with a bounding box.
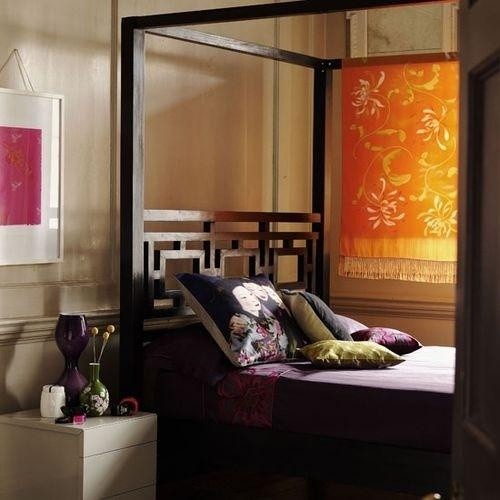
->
[120,0,500,499]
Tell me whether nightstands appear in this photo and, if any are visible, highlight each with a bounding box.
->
[0,407,157,500]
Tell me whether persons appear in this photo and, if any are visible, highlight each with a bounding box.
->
[259,279,311,360]
[239,278,288,328]
[225,280,288,364]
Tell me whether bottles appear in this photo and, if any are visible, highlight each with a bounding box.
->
[40,384,66,419]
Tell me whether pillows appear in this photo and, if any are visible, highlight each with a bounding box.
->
[333,312,368,333]
[369,325,423,357]
[296,339,406,371]
[172,271,311,367]
[280,288,353,344]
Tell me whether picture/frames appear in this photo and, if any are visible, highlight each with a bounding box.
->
[0,87,65,266]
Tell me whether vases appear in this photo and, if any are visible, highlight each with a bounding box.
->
[55,312,90,416]
[79,363,110,418]
